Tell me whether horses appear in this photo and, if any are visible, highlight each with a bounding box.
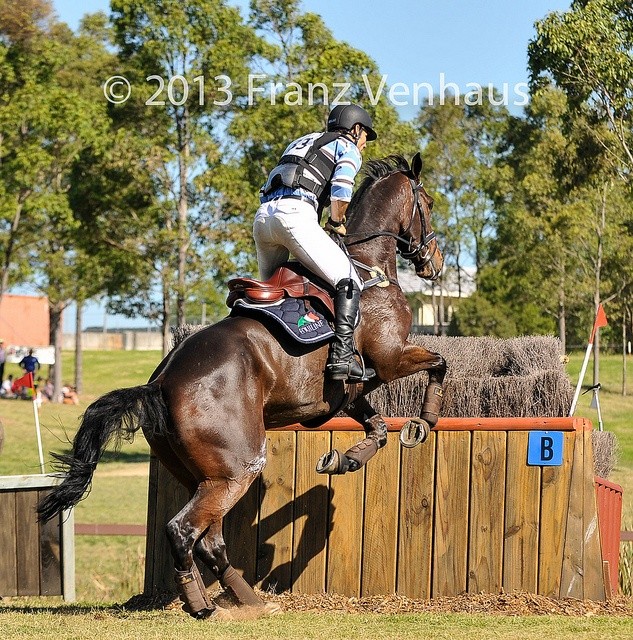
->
[33,152,446,616]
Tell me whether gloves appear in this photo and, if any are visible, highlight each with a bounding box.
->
[322,217,346,242]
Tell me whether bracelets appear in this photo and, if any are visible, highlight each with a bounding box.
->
[328,215,346,227]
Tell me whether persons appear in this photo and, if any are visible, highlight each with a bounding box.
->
[41,379,55,400]
[18,348,41,379]
[1,375,17,401]
[0,340,7,389]
[59,383,81,406]
[253,102,381,384]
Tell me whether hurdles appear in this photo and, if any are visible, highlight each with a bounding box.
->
[0,472,74,601]
[144,418,609,605]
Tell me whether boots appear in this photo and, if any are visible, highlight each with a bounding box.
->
[325,278,376,381]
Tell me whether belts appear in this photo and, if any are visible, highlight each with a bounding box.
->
[269,195,315,207]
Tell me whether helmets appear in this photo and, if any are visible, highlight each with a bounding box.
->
[327,104,377,141]
